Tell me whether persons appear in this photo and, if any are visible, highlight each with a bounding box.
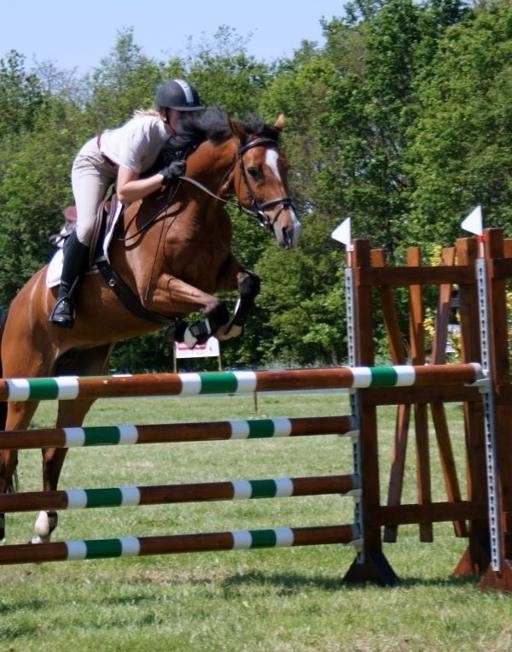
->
[53,78,206,329]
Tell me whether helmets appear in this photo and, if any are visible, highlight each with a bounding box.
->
[155,79,203,111]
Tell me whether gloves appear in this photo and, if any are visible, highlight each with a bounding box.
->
[160,161,184,186]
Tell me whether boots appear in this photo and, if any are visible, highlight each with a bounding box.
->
[51,230,88,328]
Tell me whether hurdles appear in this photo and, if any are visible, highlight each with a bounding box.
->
[0,228,511,591]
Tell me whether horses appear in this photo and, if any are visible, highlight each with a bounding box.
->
[0,105,303,546]
